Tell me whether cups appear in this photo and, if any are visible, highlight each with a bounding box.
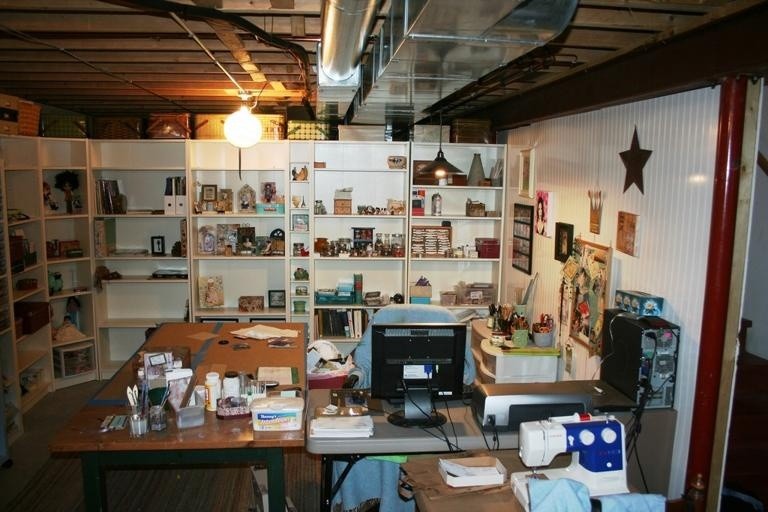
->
[124,400,149,439]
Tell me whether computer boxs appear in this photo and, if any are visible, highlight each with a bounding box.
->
[600,308,681,411]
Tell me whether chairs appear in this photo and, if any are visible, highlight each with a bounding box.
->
[343,306,478,510]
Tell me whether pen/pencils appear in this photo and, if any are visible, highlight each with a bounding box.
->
[159,383,172,407]
[488,303,531,331]
[124,381,146,421]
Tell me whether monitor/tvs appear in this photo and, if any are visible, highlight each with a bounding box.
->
[369,323,468,429]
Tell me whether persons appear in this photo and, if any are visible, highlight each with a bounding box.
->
[239,193,249,209]
[43,182,59,210]
[241,236,253,248]
[534,197,545,235]
[263,184,274,202]
[294,219,307,232]
[55,171,80,214]
[64,297,81,329]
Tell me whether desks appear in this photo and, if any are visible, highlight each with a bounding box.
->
[310,381,676,511]
[53,320,310,512]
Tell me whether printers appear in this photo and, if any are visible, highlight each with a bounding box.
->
[472,382,594,432]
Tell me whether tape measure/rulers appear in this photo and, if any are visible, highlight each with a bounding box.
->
[179,364,203,411]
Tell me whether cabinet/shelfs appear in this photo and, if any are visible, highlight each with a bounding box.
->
[408,142,505,314]
[1,137,95,453]
[90,140,189,378]
[187,141,288,325]
[470,313,560,385]
[290,140,315,343]
[313,139,406,343]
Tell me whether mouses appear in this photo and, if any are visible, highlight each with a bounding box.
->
[351,391,365,404]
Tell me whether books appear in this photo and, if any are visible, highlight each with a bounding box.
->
[96,179,119,216]
[94,219,116,257]
[162,175,186,195]
[314,308,369,339]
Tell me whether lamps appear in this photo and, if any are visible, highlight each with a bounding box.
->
[224,92,262,150]
[419,93,463,178]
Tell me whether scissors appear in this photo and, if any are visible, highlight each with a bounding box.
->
[538,327,550,334]
[541,313,553,328]
